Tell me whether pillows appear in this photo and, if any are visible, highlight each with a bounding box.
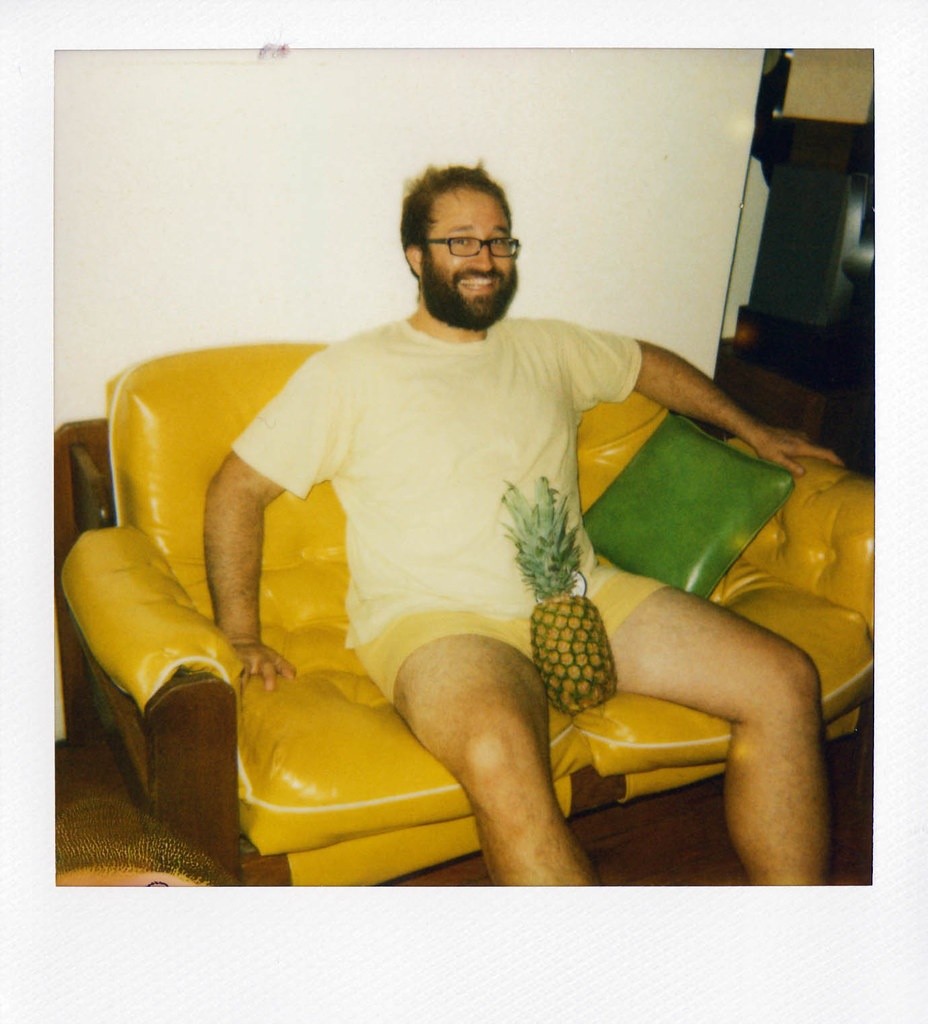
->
[584,411,794,599]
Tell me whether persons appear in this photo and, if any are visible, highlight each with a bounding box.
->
[202,164,849,885]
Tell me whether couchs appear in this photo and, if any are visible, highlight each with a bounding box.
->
[56,341,873,887]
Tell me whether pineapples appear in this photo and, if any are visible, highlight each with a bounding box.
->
[500,478,622,710]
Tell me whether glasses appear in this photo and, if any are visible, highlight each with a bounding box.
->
[427,236,519,258]
